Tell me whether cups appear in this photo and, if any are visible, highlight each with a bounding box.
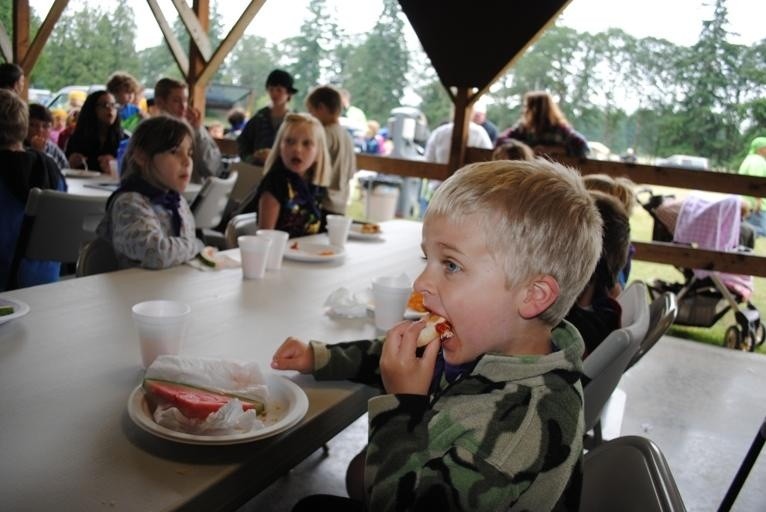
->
[132,299,190,366]
[236,234,272,282]
[109,160,118,182]
[255,229,288,270]
[327,214,352,247]
[371,275,412,333]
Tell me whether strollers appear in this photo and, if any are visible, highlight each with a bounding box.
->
[621,176,766,354]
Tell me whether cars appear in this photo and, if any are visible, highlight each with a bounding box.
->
[655,153,710,170]
[37,79,158,126]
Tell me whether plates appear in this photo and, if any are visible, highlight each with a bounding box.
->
[60,169,102,178]
[325,223,384,242]
[284,244,347,263]
[131,369,309,442]
[0,295,31,327]
[364,299,433,320]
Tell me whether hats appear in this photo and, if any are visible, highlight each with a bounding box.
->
[268,69,298,94]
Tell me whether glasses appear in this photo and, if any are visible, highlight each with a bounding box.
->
[96,102,121,108]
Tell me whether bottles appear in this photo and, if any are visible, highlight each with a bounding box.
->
[117,141,128,181]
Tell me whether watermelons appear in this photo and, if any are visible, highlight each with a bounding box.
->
[144,377,265,421]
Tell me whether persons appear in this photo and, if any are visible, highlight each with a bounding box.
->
[472,102,498,151]
[583,164,634,294]
[491,138,536,161]
[621,148,638,165]
[268,161,603,510]
[565,193,630,363]
[2,58,392,289]
[496,90,589,161]
[421,97,490,199]
[740,137,766,240]
[739,200,756,253]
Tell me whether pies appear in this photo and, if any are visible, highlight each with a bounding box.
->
[412,312,453,348]
